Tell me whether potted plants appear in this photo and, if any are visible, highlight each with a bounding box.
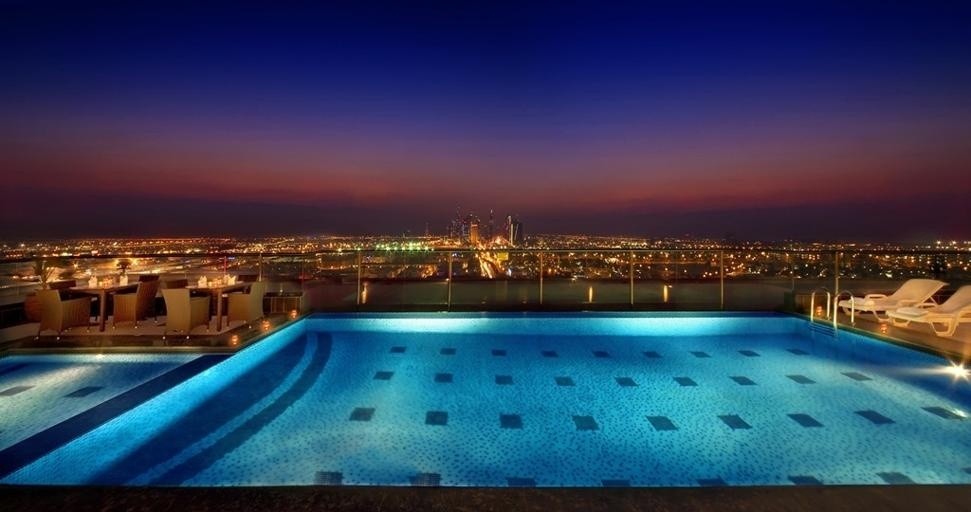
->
[24,251,64,321]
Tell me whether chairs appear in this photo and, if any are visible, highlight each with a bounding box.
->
[35,274,268,341]
[839,279,971,337]
